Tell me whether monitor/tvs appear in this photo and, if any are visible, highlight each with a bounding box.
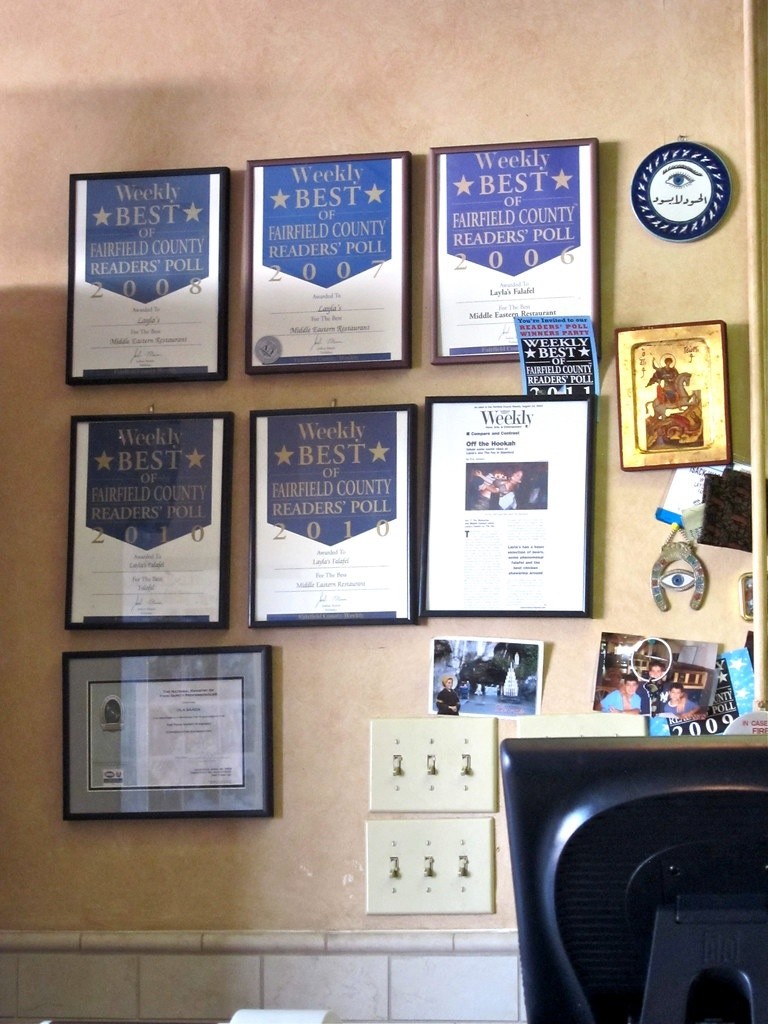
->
[499,737,768,1024]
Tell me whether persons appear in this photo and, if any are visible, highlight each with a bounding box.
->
[436,675,461,715]
[594,661,701,718]
[466,465,530,509]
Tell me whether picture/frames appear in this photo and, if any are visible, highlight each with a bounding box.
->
[617,320,737,471]
